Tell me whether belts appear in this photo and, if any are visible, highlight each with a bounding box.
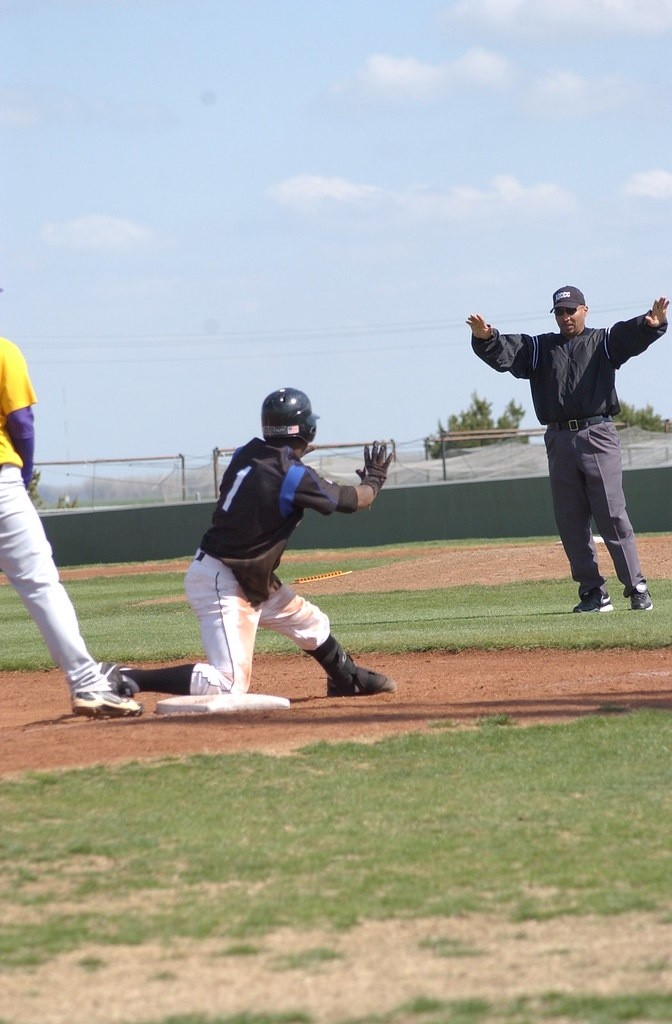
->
[547,414,613,431]
[196,552,205,561]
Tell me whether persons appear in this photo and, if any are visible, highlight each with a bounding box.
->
[98,387,397,696]
[465,286,669,610]
[0,286,142,718]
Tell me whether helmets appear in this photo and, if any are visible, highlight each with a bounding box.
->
[261,388,320,443]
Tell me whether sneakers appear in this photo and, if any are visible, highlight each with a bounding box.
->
[573,592,613,613]
[327,670,397,695]
[97,662,132,699]
[630,585,654,610]
[70,691,143,719]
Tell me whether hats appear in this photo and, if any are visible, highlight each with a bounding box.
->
[550,286,585,313]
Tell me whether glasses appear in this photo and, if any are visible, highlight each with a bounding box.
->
[553,308,582,316]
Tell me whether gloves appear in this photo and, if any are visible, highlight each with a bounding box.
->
[355,440,395,498]
